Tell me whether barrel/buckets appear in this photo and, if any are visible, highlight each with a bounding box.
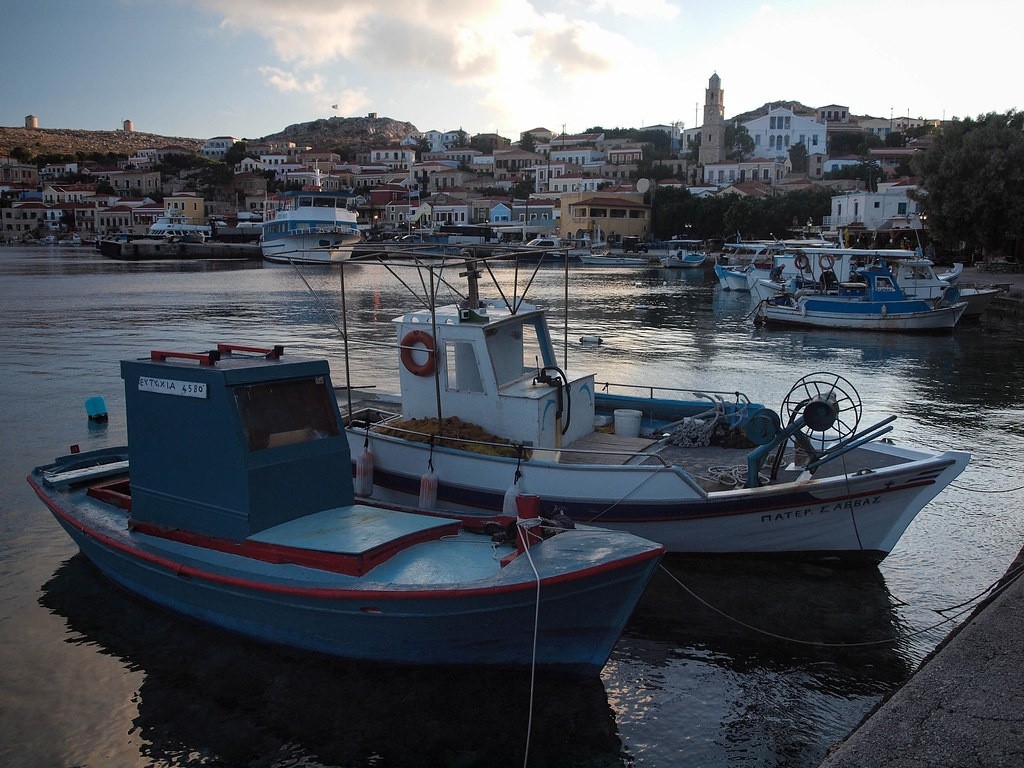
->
[614,409,642,438]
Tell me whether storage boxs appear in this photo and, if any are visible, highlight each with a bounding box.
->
[531,450,561,463]
[595,416,613,426]
[596,423,615,434]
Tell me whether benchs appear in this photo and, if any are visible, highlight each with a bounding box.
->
[997,283,1013,292]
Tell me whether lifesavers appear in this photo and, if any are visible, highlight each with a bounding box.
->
[794,255,809,271]
[400,330,437,377]
[818,254,835,270]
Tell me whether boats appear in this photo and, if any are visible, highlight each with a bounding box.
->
[24,232,83,246]
[260,159,363,264]
[27,342,668,681]
[148,215,212,243]
[264,234,971,571]
[384,231,650,266]
[762,248,970,328]
[713,230,1014,320]
[659,240,707,268]
